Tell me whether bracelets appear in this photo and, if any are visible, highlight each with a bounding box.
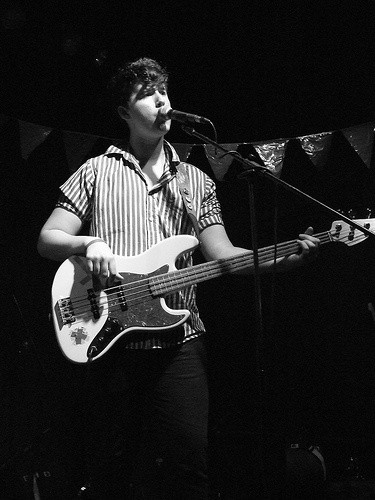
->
[85,239,106,252]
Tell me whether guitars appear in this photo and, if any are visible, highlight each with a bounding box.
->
[43,206,375,363]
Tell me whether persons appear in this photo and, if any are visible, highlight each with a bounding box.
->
[37,58,320,500]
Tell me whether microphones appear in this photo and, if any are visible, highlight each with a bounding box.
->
[158,106,210,125]
